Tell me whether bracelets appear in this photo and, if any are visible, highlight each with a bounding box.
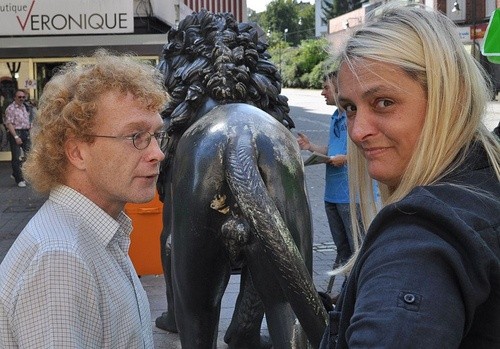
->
[14,135,18,140]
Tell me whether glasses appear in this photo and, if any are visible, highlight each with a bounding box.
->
[333,119,341,138]
[16,95,26,98]
[76,130,170,151]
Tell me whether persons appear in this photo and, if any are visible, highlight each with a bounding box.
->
[5,90,38,188]
[295,70,381,306]
[320,6,500,349]
[0,54,168,349]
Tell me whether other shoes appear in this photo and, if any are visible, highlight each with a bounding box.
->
[18,181,26,187]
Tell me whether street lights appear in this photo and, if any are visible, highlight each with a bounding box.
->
[451,0,477,59]
